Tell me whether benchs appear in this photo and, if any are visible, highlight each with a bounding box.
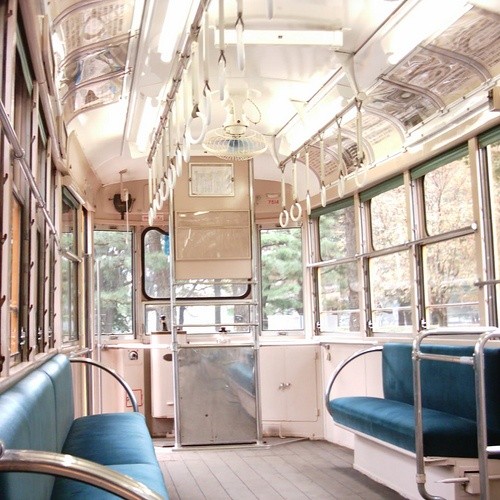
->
[0,350,167,499]
[324,341,500,500]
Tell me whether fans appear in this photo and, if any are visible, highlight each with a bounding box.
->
[202,77,269,160]
